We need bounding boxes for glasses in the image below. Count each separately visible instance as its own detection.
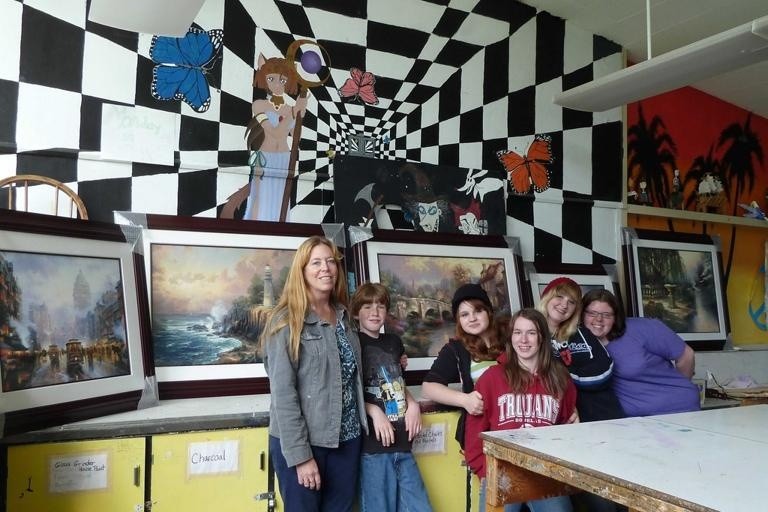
[585,310,613,319]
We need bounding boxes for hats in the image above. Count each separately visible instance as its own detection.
[452,284,489,316]
[542,277,579,296]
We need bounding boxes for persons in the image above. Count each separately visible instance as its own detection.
[349,283,432,511]
[536,278,625,421]
[243,58,309,219]
[464,308,581,512]
[260,237,408,512]
[579,288,700,417]
[422,285,505,454]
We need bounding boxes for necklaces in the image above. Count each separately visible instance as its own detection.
[518,358,539,376]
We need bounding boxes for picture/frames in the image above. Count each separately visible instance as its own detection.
[622,226,734,352]
[0,208,159,434]
[520,262,624,316]
[114,210,350,400]
[348,225,531,387]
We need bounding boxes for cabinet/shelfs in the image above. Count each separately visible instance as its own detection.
[3,435,149,511]
[148,428,273,511]
[470,472,480,511]
[410,410,469,511]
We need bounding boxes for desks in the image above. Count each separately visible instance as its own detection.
[479,402,768,511]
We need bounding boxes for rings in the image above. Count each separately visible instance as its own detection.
[310,480,315,484]
